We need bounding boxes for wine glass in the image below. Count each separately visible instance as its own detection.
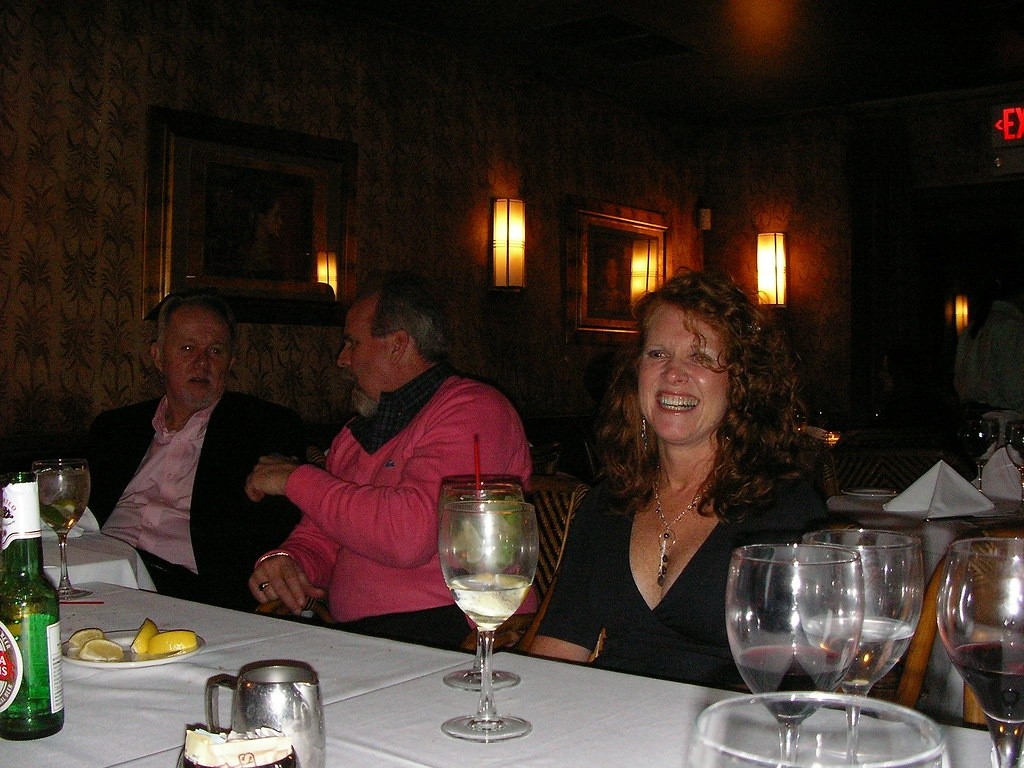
[934,538,1024,767]
[1005,421,1024,518]
[965,418,998,492]
[795,530,921,768]
[32,458,92,599]
[438,501,540,740]
[725,539,864,768]
[442,483,527,689]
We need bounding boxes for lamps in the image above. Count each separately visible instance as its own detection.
[756,231,786,308]
[488,198,530,294]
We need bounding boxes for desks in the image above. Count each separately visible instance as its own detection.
[0,531,994,768]
[821,494,1023,730]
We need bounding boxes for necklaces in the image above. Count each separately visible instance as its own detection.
[645,466,712,589]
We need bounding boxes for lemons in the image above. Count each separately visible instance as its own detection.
[40,499,76,528]
[130,619,197,655]
[450,573,529,615]
[69,628,124,660]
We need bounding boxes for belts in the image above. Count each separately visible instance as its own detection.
[139,549,178,574]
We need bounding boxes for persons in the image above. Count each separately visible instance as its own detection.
[243,286,541,652]
[85,287,305,620]
[526,265,832,698]
[945,270,1024,415]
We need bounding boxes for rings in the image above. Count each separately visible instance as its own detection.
[257,581,272,591]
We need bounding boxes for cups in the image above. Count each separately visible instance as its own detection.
[177,739,297,768]
[687,689,946,767]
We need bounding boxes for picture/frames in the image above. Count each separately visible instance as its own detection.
[140,103,358,328]
[558,190,672,346]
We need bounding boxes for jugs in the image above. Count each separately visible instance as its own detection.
[204,659,328,768]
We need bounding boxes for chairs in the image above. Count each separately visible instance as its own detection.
[462,444,1024,732]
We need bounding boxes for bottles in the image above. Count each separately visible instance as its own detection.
[0,470,64,742]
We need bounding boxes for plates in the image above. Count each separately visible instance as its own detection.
[840,488,898,498]
[61,628,206,669]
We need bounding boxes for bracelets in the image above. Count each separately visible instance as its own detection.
[257,551,290,564]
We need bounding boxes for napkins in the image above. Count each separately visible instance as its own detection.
[886,461,994,521]
